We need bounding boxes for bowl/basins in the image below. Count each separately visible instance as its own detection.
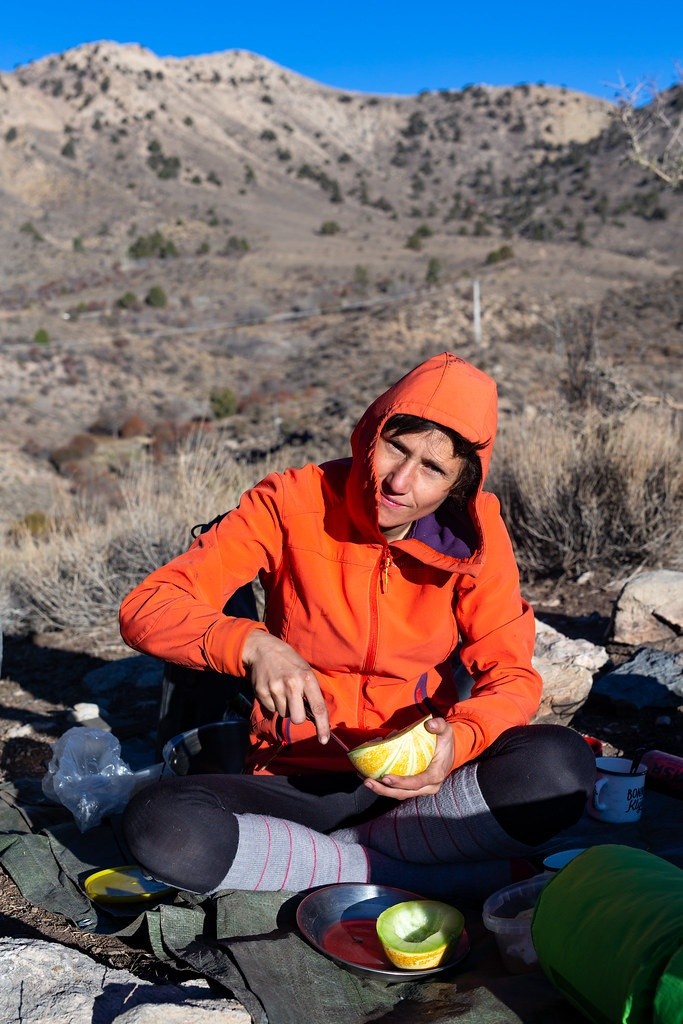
[482,873,553,971]
[162,719,250,776]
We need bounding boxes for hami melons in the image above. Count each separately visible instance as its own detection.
[376,899,470,970]
[348,713,438,781]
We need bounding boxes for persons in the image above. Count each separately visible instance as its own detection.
[119,353,598,896]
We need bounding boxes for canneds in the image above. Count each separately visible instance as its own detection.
[642,749,683,794]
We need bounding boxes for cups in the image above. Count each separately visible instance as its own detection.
[585,757,648,823]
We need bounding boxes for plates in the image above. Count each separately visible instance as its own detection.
[296,883,470,983]
[543,848,586,870]
